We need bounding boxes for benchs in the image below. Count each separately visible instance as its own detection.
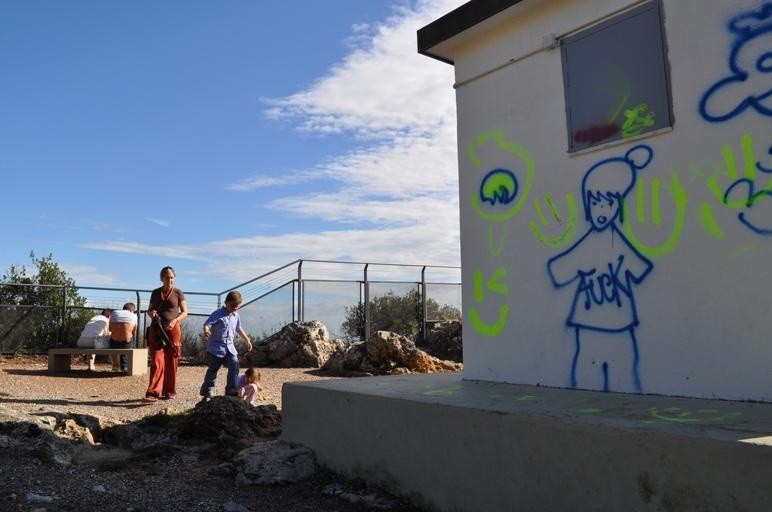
[46,347,149,376]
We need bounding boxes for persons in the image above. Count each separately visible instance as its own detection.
[77,309,113,370]
[200,291,253,401]
[238,368,263,404]
[109,303,138,374]
[142,267,188,401]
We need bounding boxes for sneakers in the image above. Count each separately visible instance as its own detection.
[89,367,128,371]
[142,393,158,402]
[165,394,175,400]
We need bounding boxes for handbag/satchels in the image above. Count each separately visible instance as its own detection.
[146,315,173,349]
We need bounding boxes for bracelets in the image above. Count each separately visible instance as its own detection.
[176,318,178,322]
